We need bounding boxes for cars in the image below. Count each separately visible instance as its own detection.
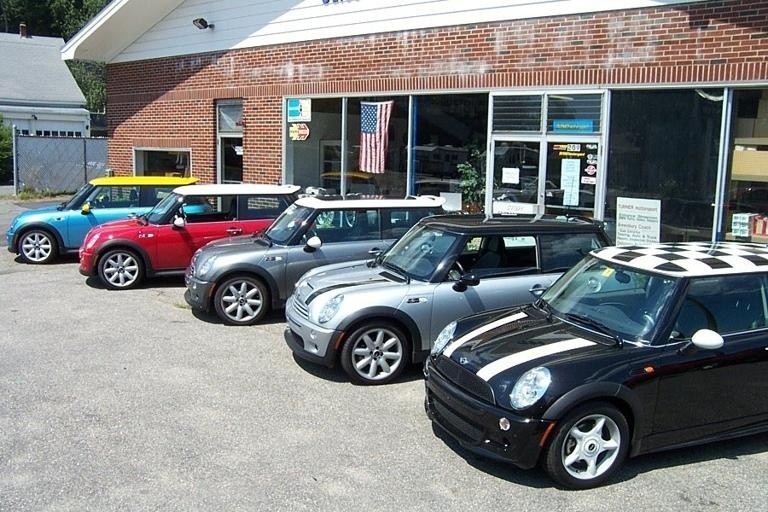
[174,195,445,327]
[422,240,767,491]
[5,176,198,265]
[316,168,573,208]
[283,210,612,386]
[79,184,297,290]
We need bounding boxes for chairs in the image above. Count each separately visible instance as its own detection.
[128,189,139,208]
[476,235,504,269]
[630,279,682,329]
[222,199,237,222]
[342,209,369,242]
[715,281,756,334]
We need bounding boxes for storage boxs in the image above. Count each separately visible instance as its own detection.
[731,137,768,183]
[735,88,768,138]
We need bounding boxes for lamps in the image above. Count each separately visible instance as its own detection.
[193,17,215,30]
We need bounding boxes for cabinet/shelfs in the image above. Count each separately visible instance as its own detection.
[319,140,341,195]
